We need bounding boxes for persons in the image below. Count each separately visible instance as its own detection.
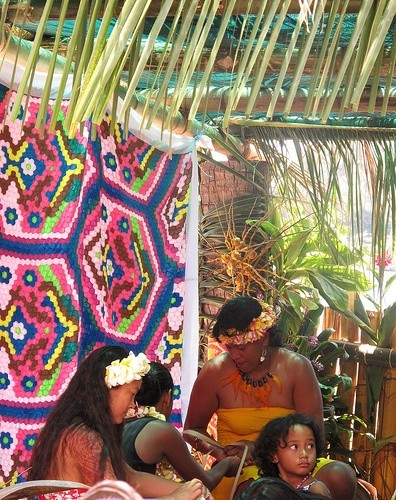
[181,294,358,500]
[120,361,244,492]
[27,344,213,500]
[251,412,332,500]
[235,474,332,500]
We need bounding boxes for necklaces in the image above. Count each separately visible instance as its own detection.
[123,401,176,484]
[295,472,312,490]
[219,348,282,409]
